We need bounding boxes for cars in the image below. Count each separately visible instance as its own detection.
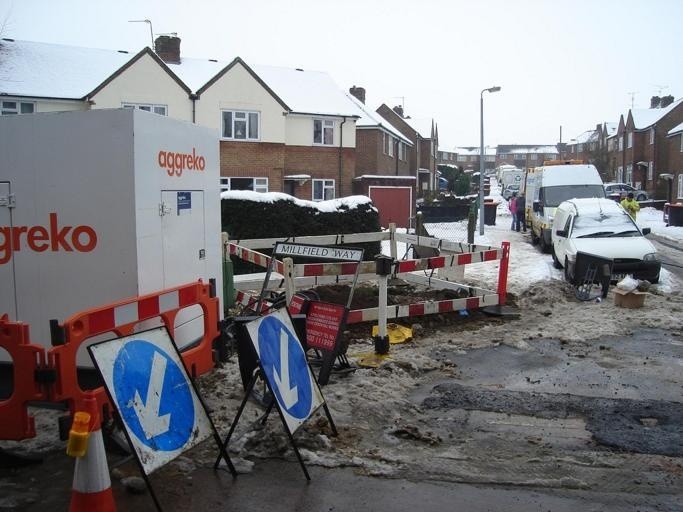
[493,161,524,198]
[600,180,645,201]
[551,198,660,285]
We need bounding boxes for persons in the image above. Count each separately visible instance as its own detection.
[620,193,640,222]
[509,192,517,230]
[516,192,527,232]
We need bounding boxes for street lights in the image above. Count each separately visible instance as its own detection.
[478,84,500,237]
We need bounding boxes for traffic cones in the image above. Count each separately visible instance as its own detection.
[67,391,116,512]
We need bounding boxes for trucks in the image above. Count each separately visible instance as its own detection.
[1,110,220,452]
[525,158,605,252]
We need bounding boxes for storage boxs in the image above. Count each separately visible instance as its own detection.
[610,286,650,307]
